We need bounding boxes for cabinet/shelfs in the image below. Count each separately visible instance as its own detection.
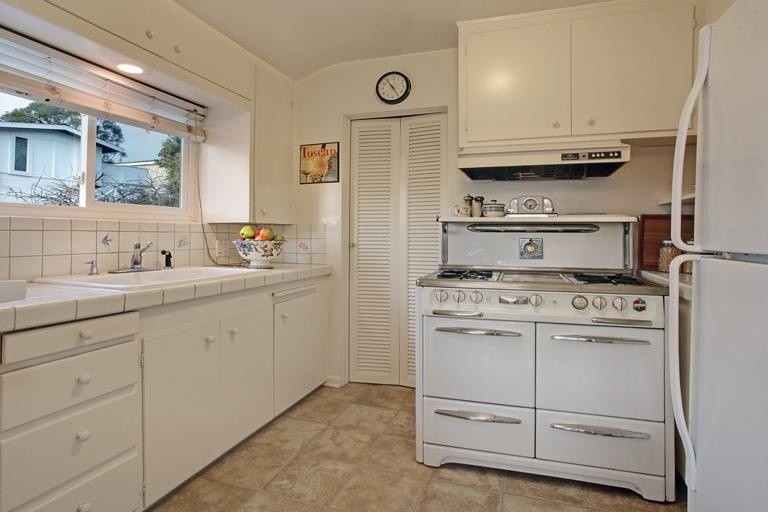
[275,277,332,422]
[458,2,699,147]
[0,311,144,512]
[144,290,276,509]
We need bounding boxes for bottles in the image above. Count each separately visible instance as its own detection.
[458,195,484,218]
[657,240,683,273]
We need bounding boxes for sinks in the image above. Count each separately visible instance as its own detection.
[31,267,256,289]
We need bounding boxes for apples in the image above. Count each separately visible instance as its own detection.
[239,225,284,241]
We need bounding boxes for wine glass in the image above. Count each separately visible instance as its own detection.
[231,240,288,271]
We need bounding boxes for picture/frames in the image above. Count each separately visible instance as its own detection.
[300,141,339,183]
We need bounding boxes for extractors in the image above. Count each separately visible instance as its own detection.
[457,142,633,181]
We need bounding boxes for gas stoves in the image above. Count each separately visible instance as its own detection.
[416,267,663,316]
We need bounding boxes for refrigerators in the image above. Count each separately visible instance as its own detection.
[664,0,768,511]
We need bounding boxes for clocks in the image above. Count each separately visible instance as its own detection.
[375,69,411,104]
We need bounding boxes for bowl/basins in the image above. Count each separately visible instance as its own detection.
[482,199,506,217]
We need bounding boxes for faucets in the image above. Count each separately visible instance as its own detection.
[132,241,154,267]
